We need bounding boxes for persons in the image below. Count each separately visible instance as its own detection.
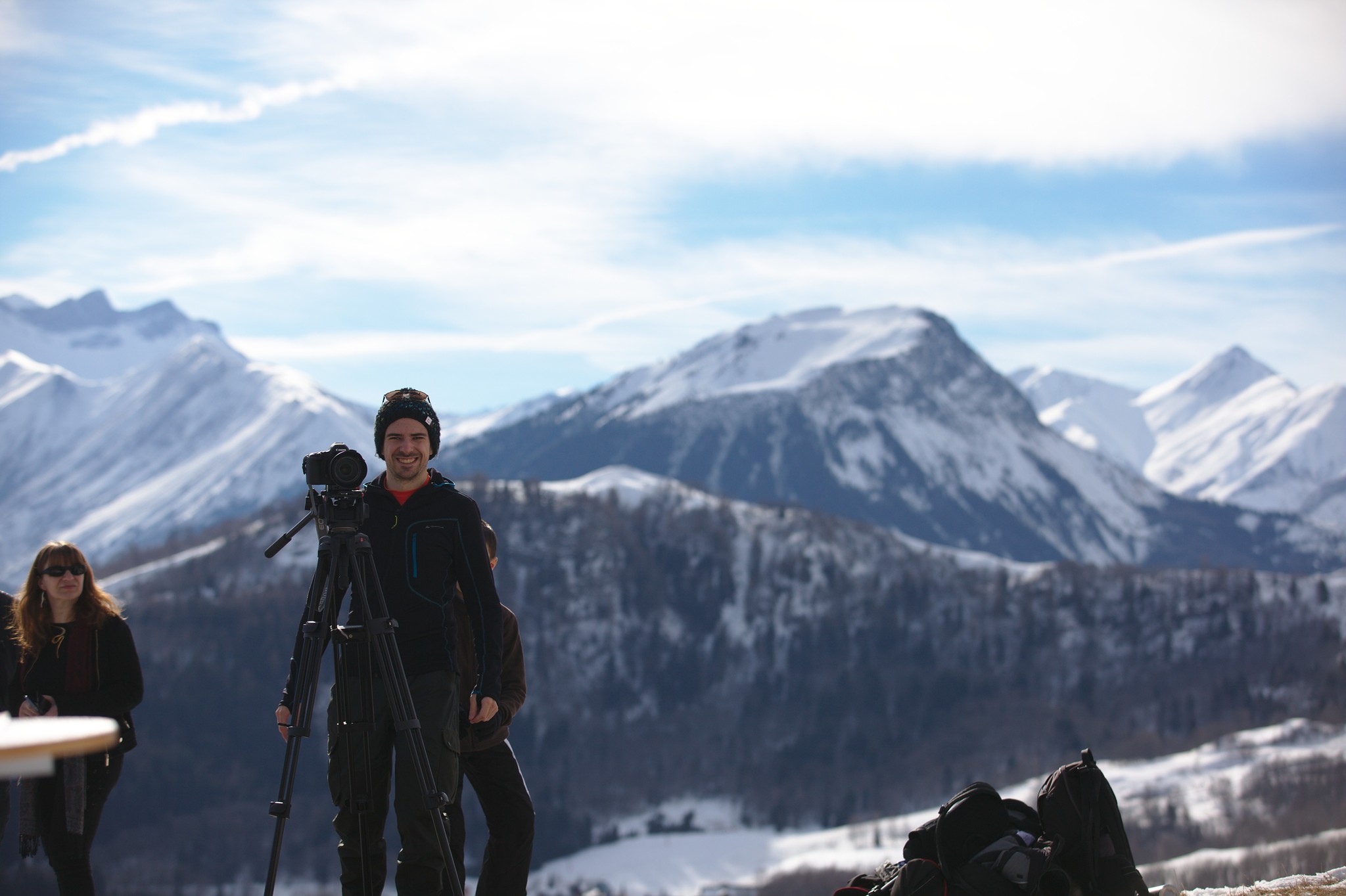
[273,387,510,896]
[414,517,539,896]
[3,539,146,896]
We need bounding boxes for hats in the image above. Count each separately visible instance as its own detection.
[374,387,440,460]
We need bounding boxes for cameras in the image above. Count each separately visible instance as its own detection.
[301,441,368,489]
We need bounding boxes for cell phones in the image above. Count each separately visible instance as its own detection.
[24,690,52,716]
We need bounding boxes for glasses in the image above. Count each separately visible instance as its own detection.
[38,563,85,577]
[382,390,432,408]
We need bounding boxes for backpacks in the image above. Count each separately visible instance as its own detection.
[835,748,1149,896]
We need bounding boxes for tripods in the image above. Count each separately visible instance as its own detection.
[262,492,466,896]
